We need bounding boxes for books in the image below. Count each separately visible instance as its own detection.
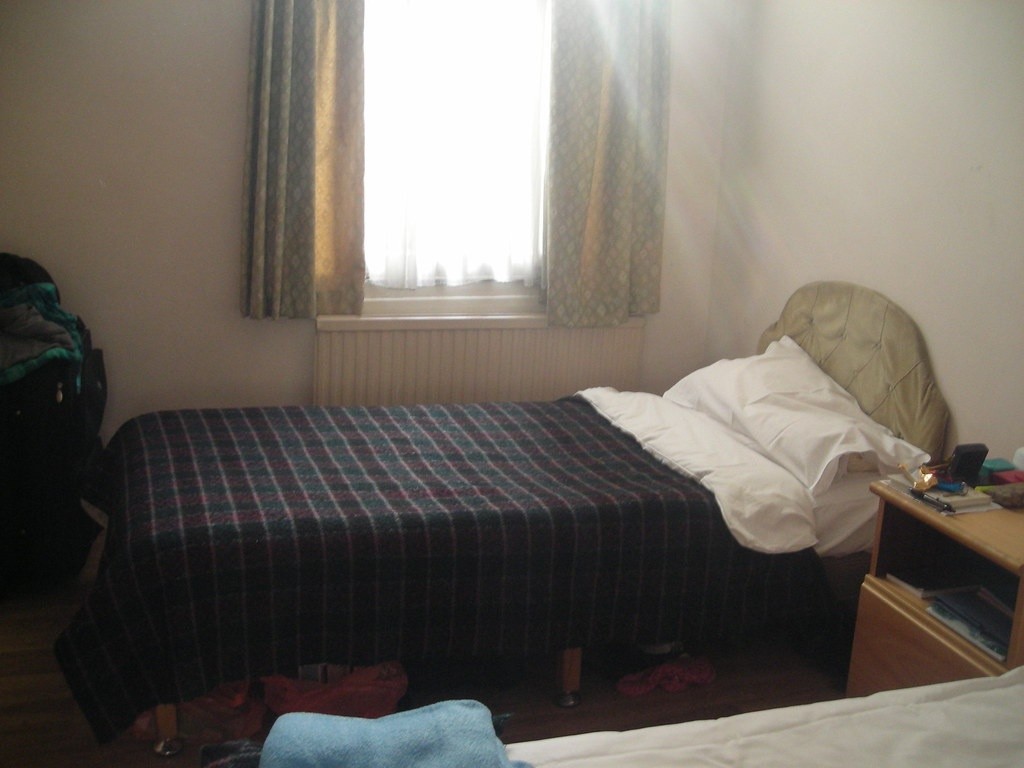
[886,557,989,599]
[924,580,1018,664]
[887,474,991,508]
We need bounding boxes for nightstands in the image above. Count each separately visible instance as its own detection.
[845,478,1024,701]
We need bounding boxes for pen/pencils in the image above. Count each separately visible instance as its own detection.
[908,487,957,513]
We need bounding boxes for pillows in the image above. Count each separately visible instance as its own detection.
[661,336,931,496]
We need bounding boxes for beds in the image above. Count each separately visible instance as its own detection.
[53,281,951,747]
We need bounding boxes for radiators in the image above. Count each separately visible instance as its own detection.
[311,313,645,403]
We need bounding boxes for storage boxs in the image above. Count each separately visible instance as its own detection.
[979,457,1015,485]
[948,444,989,481]
